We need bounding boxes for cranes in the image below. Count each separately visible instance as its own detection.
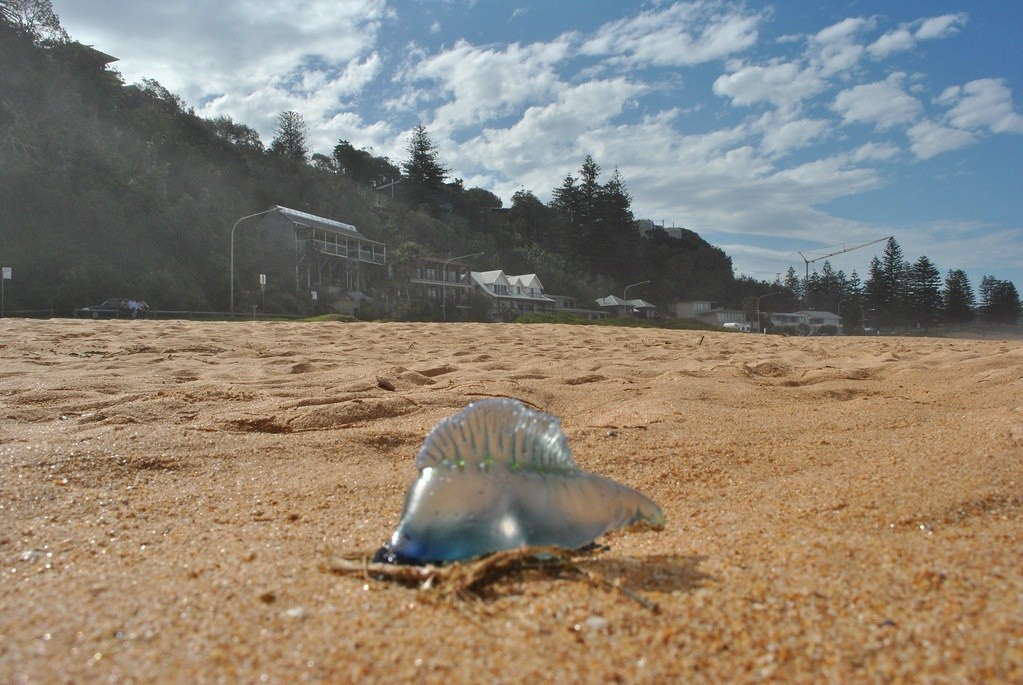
[797,235,892,311]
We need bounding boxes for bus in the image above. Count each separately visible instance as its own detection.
[724,322,753,333]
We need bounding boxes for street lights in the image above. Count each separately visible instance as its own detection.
[230,205,283,314]
[623,279,651,315]
[441,250,487,321]
[756,291,781,331]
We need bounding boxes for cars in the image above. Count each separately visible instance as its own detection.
[75,297,132,318]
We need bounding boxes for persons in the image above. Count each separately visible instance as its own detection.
[138,299,151,319]
[129,296,140,319]
[120,297,132,319]
